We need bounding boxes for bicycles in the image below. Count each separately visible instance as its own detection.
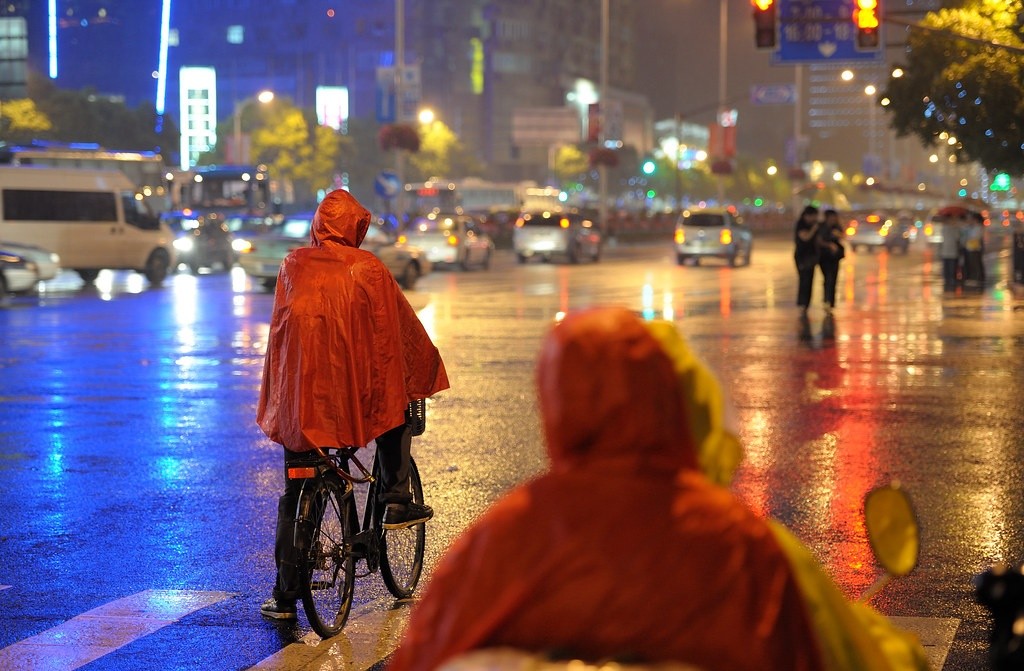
[287,398,425,638]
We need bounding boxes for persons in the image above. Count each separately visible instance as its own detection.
[943,211,984,292]
[257,188,450,618]
[387,306,930,671]
[794,206,844,309]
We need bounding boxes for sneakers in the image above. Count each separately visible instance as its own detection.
[384,502,433,529]
[262,599,296,618]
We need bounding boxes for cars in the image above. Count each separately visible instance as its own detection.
[511,212,602,264]
[844,212,943,254]
[0,241,59,299]
[159,212,432,290]
[396,216,495,272]
[674,207,752,268]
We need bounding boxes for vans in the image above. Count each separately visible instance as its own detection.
[0,182,174,284]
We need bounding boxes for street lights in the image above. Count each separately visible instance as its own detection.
[232,88,274,163]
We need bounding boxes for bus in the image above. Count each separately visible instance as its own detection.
[0,147,276,239]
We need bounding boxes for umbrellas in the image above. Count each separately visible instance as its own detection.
[939,196,991,214]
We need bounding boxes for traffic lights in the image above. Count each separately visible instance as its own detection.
[853,0,881,53]
[993,173,1010,201]
[642,159,659,179]
[753,0,776,48]
